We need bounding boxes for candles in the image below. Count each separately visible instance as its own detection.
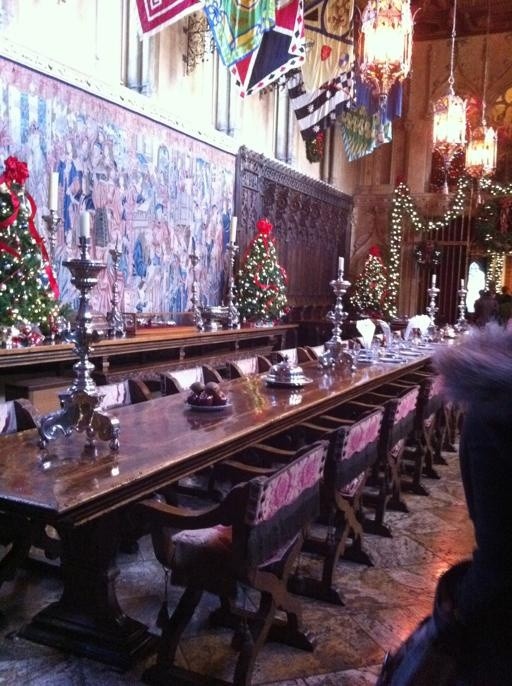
[460,278,465,287]
[229,213,238,243]
[48,167,60,210]
[80,209,91,240]
[431,272,437,284]
[338,254,345,272]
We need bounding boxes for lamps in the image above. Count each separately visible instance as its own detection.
[428,0,497,180]
[360,0,418,109]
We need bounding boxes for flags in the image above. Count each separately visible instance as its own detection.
[134,0,415,164]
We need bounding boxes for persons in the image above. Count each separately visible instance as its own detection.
[471,284,511,329]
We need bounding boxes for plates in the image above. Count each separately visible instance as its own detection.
[183,401,233,411]
[347,325,458,365]
[260,374,314,387]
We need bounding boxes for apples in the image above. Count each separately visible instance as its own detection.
[187,381,229,407]
